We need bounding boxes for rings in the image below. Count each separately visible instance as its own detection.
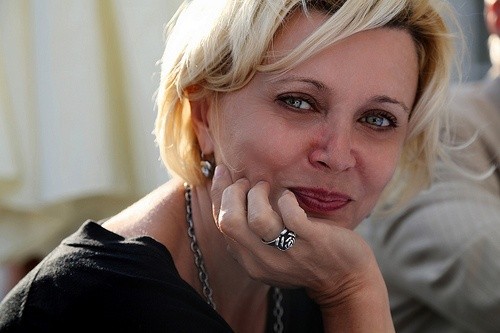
[260,225,297,253]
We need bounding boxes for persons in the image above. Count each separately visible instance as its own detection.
[347,0,498,332]
[0,0,464,333]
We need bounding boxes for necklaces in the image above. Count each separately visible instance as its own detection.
[181,182,284,333]
